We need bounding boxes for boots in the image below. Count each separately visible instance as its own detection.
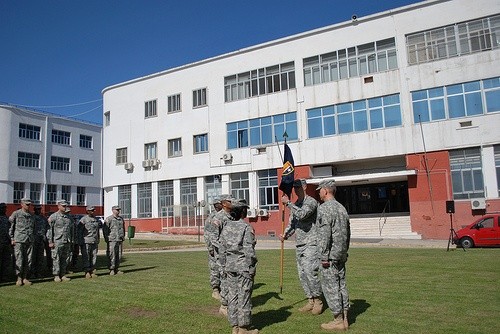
[312,297,323,315]
[212,288,220,300]
[343,310,349,330]
[231,325,239,334]
[219,305,229,316]
[238,326,259,334]
[321,314,345,330]
[297,298,315,312]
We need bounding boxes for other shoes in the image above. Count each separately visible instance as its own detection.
[109,269,123,275]
[85,271,98,279]
[54,275,71,282]
[15,276,32,286]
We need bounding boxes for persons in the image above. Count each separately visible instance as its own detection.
[46,199,75,282]
[203,194,248,317]
[0,203,80,281]
[219,200,259,334]
[279,178,323,316]
[7,197,37,285]
[103,206,125,276]
[76,205,100,278]
[316,179,350,330]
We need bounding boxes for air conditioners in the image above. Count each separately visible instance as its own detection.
[470,198,487,210]
[223,152,232,161]
[142,160,150,168]
[246,209,258,218]
[124,163,133,171]
[257,209,269,217]
[150,158,160,167]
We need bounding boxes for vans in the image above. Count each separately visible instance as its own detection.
[452,214,500,249]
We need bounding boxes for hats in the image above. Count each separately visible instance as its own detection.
[65,208,71,212]
[86,206,95,211]
[231,198,250,209]
[57,199,68,206]
[112,206,121,210]
[21,198,31,205]
[34,205,42,209]
[213,198,222,205]
[0,202,7,208]
[293,178,306,187]
[220,194,234,202]
[316,179,336,190]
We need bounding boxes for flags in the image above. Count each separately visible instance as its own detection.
[279,142,295,200]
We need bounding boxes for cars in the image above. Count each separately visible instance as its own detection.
[95,216,105,227]
